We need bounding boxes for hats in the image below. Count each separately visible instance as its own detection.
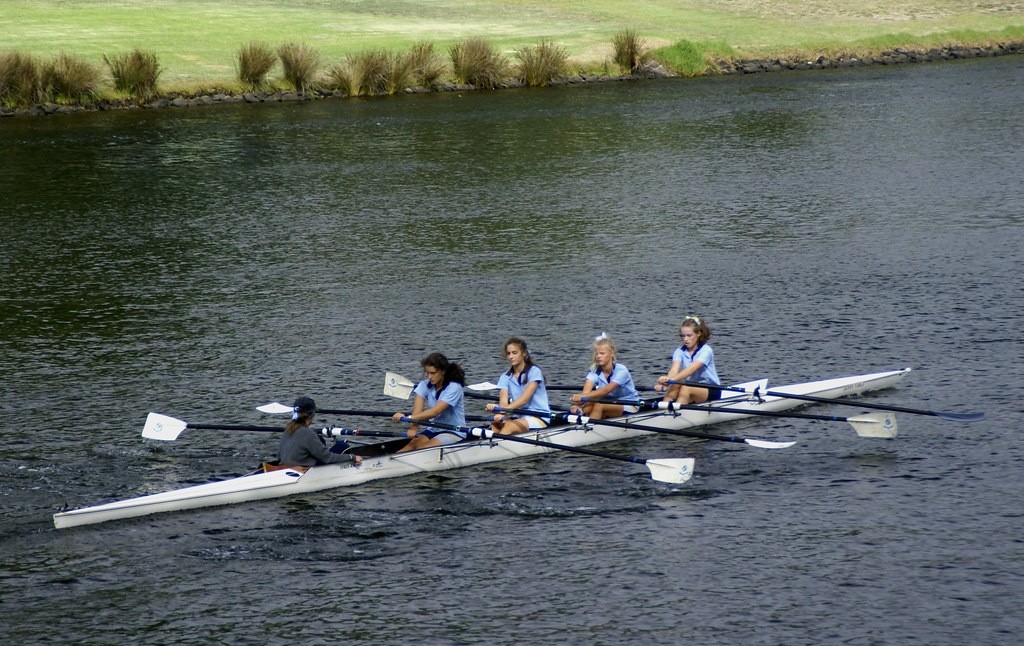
[294,396,315,413]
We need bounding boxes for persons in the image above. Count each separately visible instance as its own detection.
[485,337,551,435]
[570,332,640,420]
[392,352,467,453]
[278,396,363,467]
[653,316,721,405]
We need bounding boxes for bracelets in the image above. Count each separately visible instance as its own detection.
[409,415,412,418]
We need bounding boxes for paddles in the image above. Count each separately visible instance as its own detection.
[669,379,985,422]
[494,405,797,450]
[421,416,696,485]
[463,375,655,398]
[140,410,410,448]
[588,397,899,441]
[378,371,492,412]
[255,402,475,438]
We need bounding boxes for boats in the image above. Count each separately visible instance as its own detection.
[52,366,912,530]
